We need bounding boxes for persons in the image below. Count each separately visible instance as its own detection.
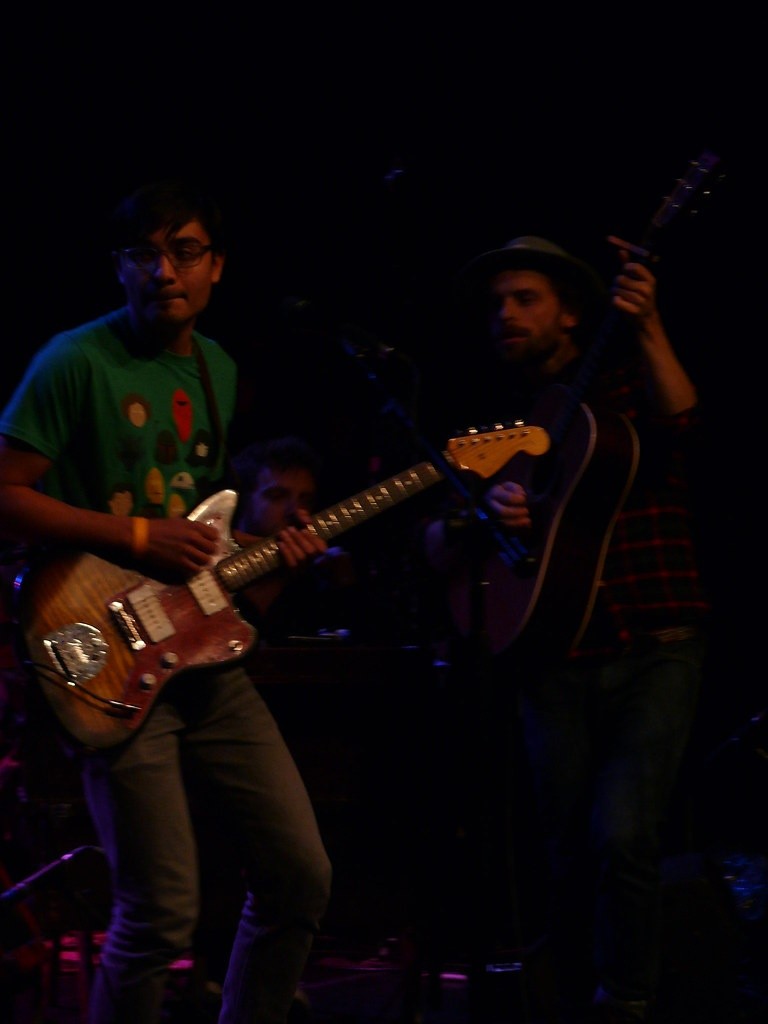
[0,192,365,1019]
[424,222,703,1023]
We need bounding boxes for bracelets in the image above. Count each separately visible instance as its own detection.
[133,517,149,554]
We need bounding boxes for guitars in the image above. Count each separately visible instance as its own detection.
[393,140,744,690]
[9,409,576,764]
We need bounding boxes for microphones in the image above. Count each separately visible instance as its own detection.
[285,297,394,359]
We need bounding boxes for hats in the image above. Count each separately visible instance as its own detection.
[468,235,604,308]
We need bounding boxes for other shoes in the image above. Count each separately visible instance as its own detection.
[609,1005,645,1024]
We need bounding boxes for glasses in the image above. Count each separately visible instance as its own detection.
[122,243,212,270]
